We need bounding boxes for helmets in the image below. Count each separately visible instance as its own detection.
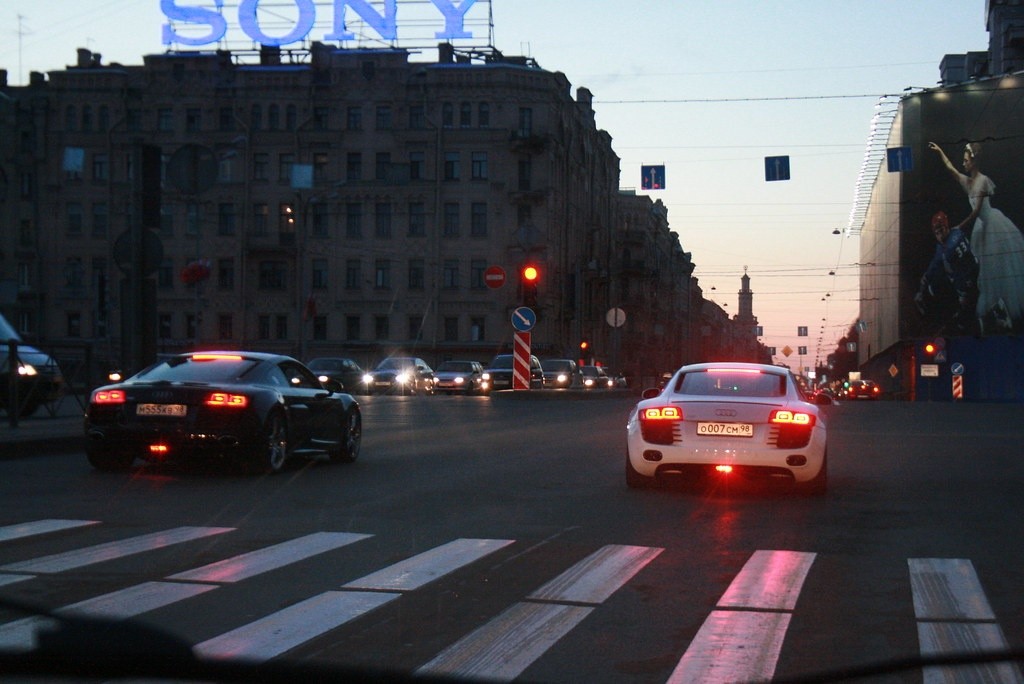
[931,211,948,233]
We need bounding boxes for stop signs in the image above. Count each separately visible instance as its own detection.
[483,265,507,289]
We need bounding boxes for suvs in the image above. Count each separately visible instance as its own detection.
[482,353,545,396]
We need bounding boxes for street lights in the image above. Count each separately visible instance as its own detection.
[298,180,348,363]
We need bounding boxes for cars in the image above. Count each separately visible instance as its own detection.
[539,358,577,390]
[579,365,627,390]
[798,375,881,406]
[363,356,436,396]
[427,360,483,396]
[306,356,365,395]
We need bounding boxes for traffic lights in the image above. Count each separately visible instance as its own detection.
[521,257,541,284]
[926,343,937,356]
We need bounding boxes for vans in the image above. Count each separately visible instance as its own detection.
[0,314,68,421]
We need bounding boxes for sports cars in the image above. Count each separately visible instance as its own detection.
[82,350,363,477]
[624,361,833,496]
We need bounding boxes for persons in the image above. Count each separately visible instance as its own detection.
[927,138,1024,331]
[914,210,1014,337]
